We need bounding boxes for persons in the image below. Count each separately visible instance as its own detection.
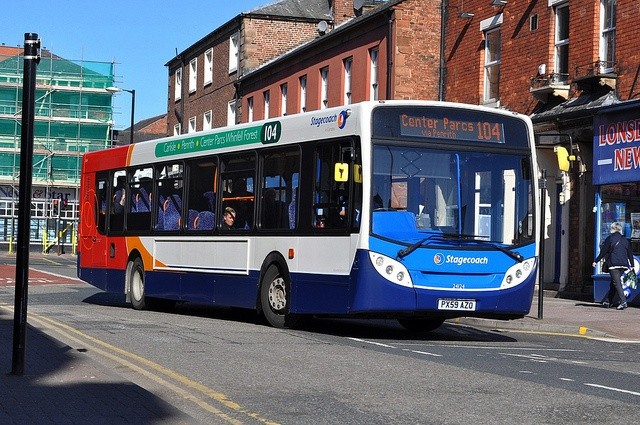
[222,208,239,233]
[593,221,635,310]
[260,189,285,229]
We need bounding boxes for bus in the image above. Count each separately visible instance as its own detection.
[76,99,541,329]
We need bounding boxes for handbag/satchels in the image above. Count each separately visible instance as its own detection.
[601,235,624,271]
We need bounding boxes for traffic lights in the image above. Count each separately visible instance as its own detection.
[54,200,58,207]
[62,199,67,205]
